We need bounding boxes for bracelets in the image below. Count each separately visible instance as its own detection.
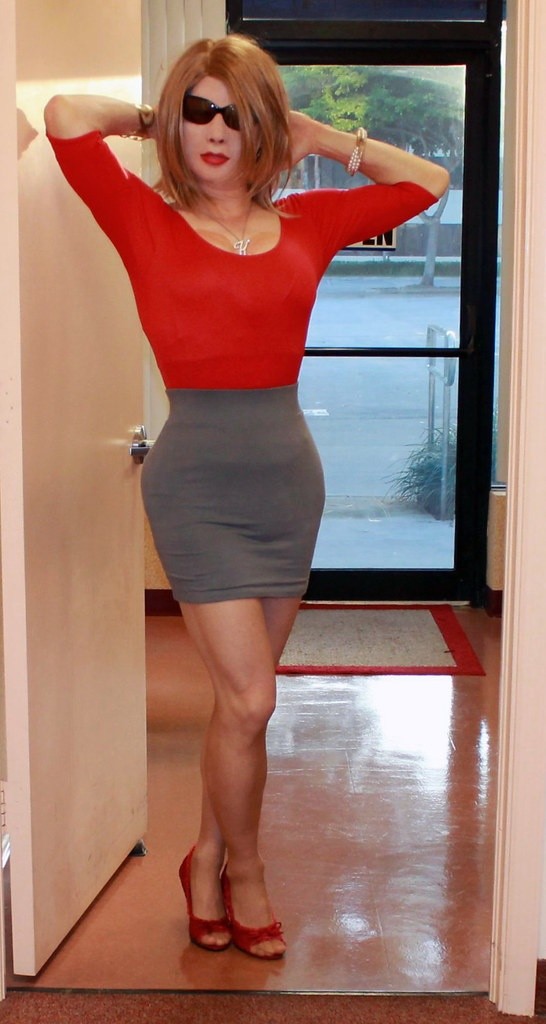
[345,126,368,177]
[119,103,142,139]
[135,102,155,140]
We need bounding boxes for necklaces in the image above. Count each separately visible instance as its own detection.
[200,203,252,256]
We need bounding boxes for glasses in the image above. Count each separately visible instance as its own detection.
[182,93,260,131]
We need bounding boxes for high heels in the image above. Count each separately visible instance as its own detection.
[221,862,287,960]
[178,845,232,952]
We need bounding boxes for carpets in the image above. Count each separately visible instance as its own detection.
[275,604,485,676]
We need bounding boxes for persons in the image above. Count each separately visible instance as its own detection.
[44,36,449,961]
